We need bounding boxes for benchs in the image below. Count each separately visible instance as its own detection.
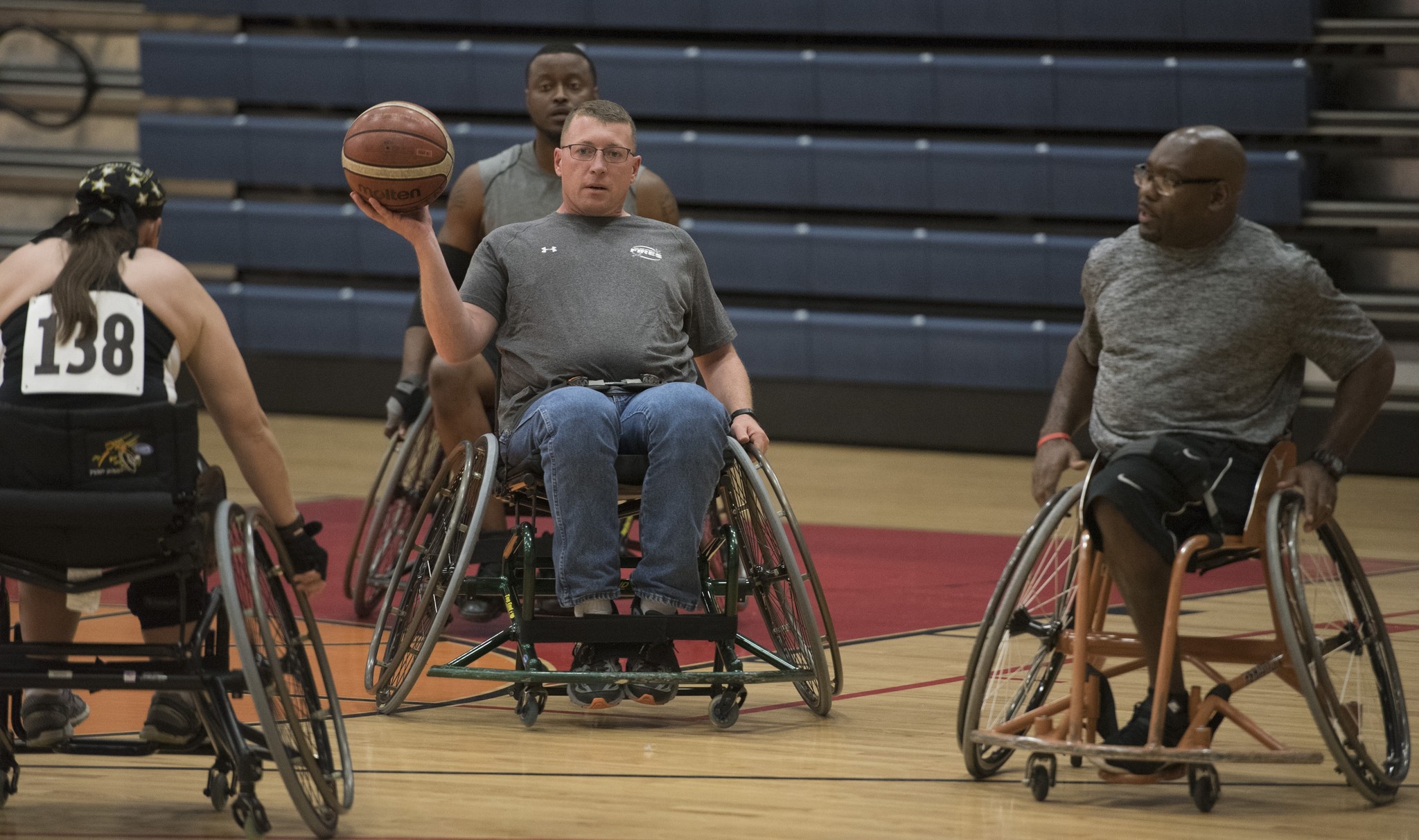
[138,0,1317,397]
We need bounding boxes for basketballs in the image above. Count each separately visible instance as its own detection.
[341,101,455,213]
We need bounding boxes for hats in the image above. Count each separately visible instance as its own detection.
[73,161,166,219]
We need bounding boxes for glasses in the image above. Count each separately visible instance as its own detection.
[1131,163,1226,195]
[560,144,637,163]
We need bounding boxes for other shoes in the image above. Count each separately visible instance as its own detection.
[458,563,506,619]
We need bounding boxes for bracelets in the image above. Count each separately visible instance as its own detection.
[728,407,758,424]
[1036,432,1072,448]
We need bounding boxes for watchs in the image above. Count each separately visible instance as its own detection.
[1309,447,1347,479]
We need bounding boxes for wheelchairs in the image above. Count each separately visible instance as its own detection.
[343,353,844,729]
[0,398,355,840]
[955,416,1412,813]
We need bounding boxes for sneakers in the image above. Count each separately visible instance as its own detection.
[623,595,682,706]
[566,599,625,709]
[18,687,89,747]
[136,690,200,748]
[1082,685,1191,773]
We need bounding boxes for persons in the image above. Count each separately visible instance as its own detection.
[1,163,328,745]
[348,43,770,708]
[1027,125,1398,777]
